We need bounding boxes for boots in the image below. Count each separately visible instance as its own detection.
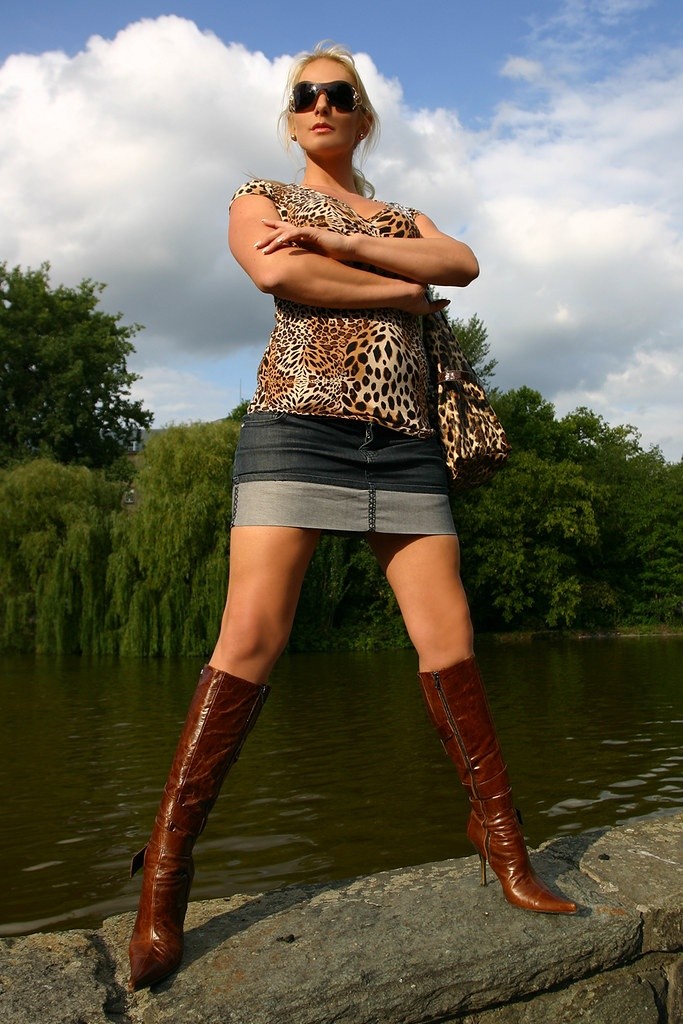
[126,664,269,988]
[418,655,578,916]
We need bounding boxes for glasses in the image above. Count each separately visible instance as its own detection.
[289,80,363,114]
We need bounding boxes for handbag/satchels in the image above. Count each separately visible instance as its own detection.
[422,284,511,494]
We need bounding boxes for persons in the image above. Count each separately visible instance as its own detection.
[122,46,576,993]
